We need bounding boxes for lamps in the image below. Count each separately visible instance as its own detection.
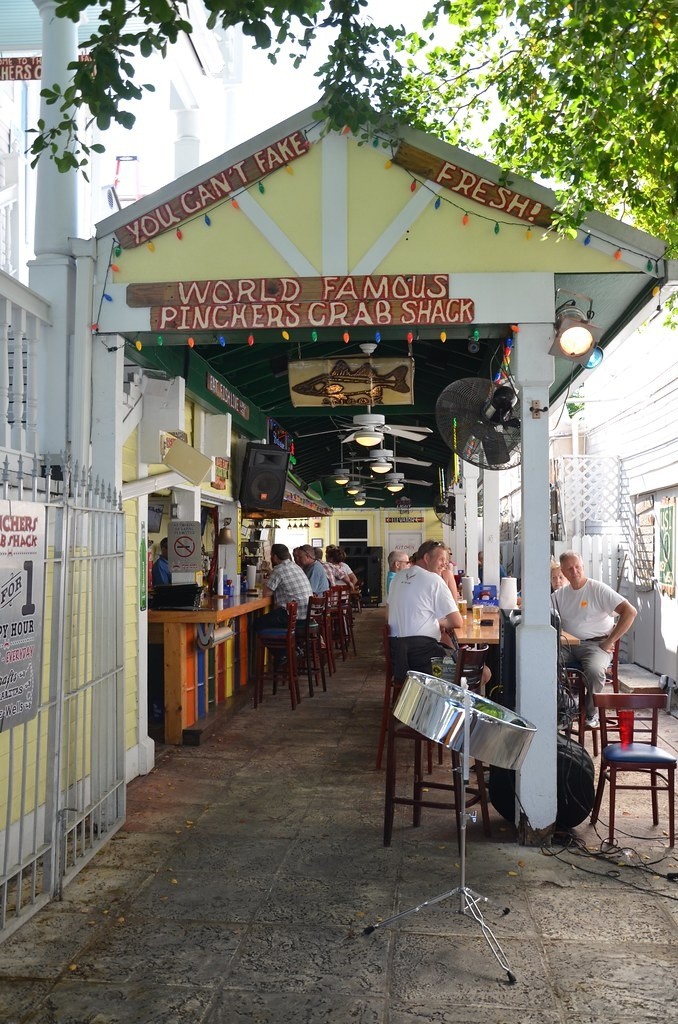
[369,462,393,473]
[548,289,605,370]
[354,499,366,506]
[334,476,349,484]
[354,432,385,447]
[387,483,404,492]
[347,487,359,495]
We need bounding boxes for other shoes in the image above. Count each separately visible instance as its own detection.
[296,657,304,668]
[274,663,289,673]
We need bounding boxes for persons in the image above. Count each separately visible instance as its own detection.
[478,551,505,584]
[254,543,358,665]
[152,537,171,586]
[387,542,492,691]
[551,550,637,728]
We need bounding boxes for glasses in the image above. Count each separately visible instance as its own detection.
[427,541,441,553]
[298,546,314,559]
[399,561,410,564]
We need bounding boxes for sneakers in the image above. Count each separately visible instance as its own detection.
[584,713,599,729]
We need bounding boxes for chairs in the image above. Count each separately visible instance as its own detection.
[590,692,677,847]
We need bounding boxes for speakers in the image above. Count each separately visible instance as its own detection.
[239,442,291,510]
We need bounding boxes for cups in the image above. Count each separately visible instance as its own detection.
[457,600,467,619]
[473,605,483,625]
[618,710,634,745]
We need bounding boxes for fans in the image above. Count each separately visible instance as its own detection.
[435,377,522,470]
[433,491,456,531]
[297,343,433,500]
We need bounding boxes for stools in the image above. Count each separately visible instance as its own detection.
[251,579,622,847]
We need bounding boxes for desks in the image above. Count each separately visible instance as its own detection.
[455,608,580,647]
